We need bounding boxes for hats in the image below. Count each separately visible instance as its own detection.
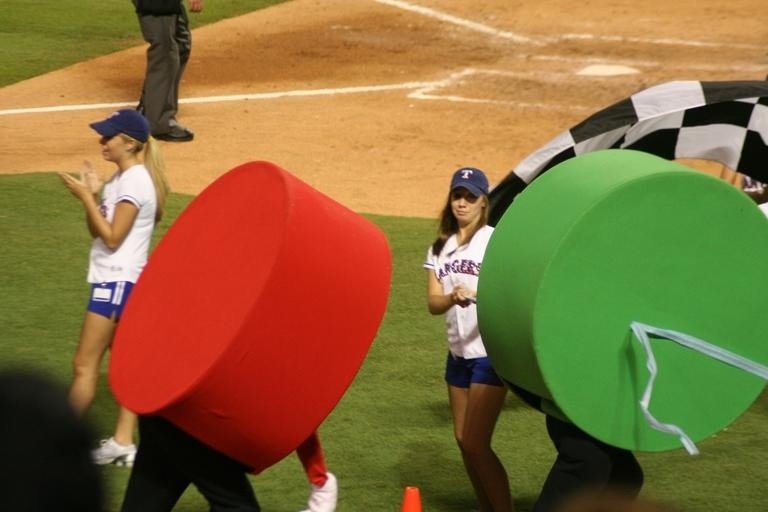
[87,108,150,143]
[450,167,489,198]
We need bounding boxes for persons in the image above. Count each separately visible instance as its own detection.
[128,1,204,142]
[54,108,171,467]
[421,166,516,510]
[118,406,261,511]
[0,366,114,510]
[536,413,644,508]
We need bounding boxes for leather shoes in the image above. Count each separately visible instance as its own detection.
[152,119,194,142]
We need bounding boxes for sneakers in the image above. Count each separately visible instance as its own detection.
[90,436,138,469]
